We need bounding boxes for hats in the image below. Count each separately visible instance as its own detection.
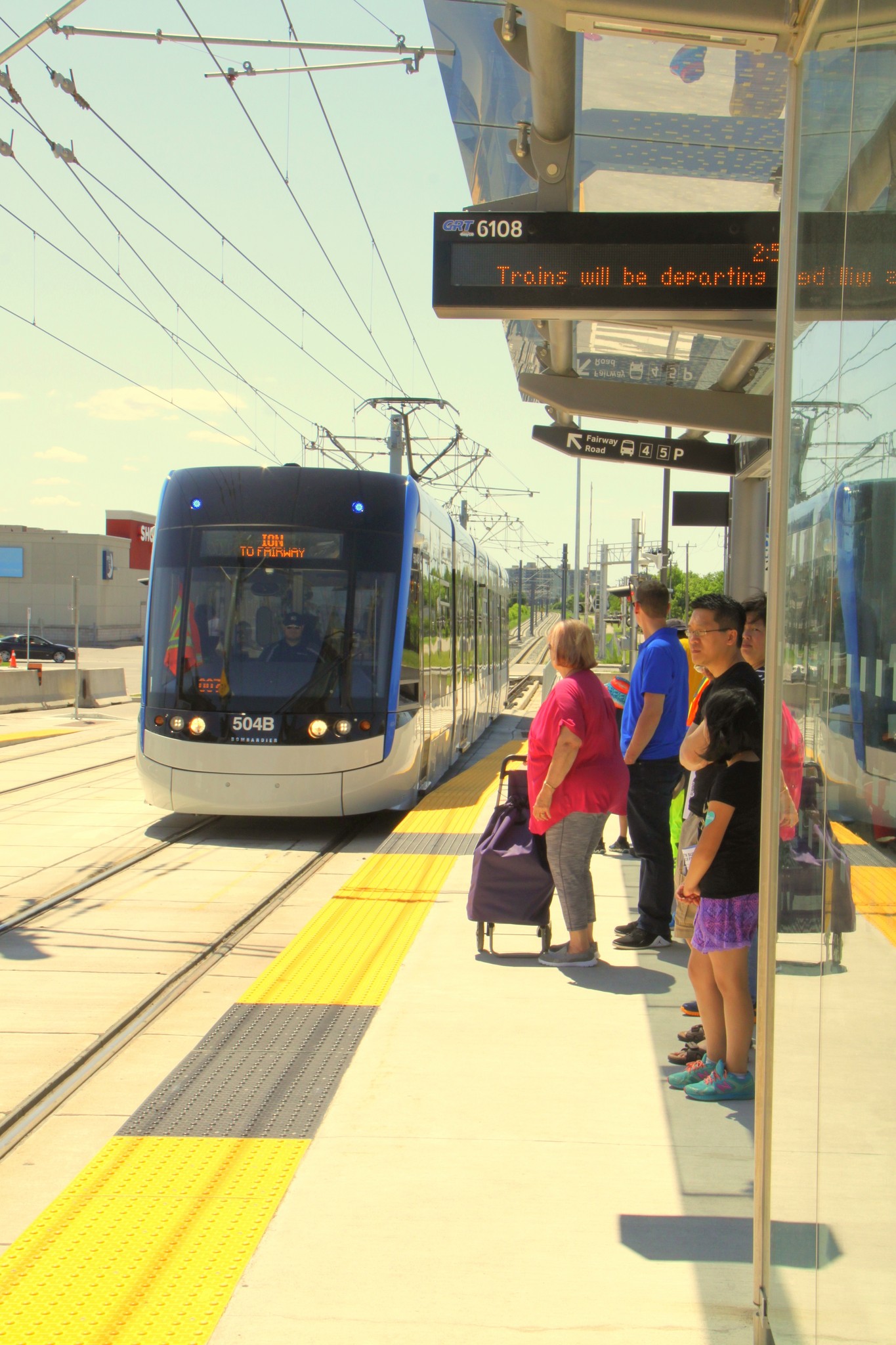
[283,612,304,627]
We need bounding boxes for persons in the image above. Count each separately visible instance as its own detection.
[667,593,764,1066]
[259,611,331,664]
[613,578,690,950]
[780,700,805,854]
[527,619,630,965]
[590,674,631,854]
[674,595,768,1024]
[665,619,704,785]
[668,685,756,1102]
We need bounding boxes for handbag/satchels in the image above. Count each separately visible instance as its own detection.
[466,793,554,926]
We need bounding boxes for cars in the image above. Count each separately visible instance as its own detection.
[0,633,77,663]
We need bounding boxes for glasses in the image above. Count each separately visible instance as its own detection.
[684,628,732,637]
[286,625,301,630]
[632,601,642,607]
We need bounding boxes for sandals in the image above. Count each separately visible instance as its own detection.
[677,1025,706,1043]
[668,1042,707,1065]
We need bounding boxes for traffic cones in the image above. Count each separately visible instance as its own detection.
[8,650,19,668]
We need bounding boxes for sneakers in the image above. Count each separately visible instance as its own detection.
[614,919,639,936]
[667,1053,716,1089]
[683,1059,755,1101]
[612,928,672,950]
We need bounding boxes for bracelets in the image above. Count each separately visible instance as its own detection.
[780,786,788,794]
[544,781,557,790]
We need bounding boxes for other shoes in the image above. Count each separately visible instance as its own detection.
[538,946,597,967]
[548,940,601,958]
[681,1001,700,1017]
[669,911,675,931]
[753,1007,757,1024]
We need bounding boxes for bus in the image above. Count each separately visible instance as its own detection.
[139,392,513,817]
